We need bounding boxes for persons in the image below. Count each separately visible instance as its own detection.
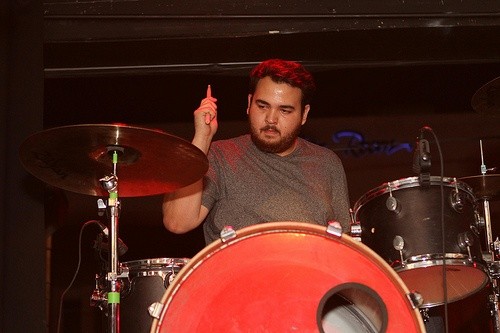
[163,58,351,249]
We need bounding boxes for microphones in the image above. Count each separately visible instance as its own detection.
[407,128,426,176]
[96,220,128,257]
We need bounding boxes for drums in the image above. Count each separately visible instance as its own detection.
[148,220,426,333]
[118,257,193,333]
[350,175,490,309]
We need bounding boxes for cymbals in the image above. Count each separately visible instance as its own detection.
[471,75,500,110]
[19,123,209,198]
